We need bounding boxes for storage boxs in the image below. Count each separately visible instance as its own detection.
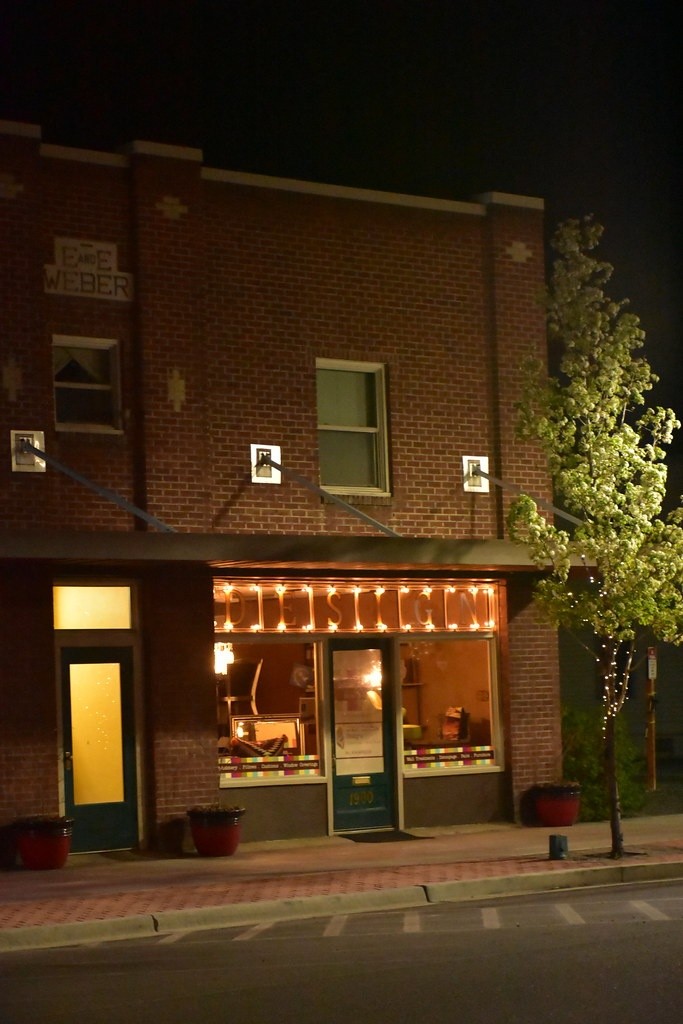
[304,721,317,755]
[299,697,316,721]
[438,711,470,741]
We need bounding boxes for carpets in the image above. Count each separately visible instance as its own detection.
[337,829,435,843]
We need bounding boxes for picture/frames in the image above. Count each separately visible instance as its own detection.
[229,713,302,754]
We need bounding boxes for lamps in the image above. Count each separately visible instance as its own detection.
[214,642,235,676]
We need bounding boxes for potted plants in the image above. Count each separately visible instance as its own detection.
[9,814,77,870]
[529,778,581,828]
[187,801,246,858]
